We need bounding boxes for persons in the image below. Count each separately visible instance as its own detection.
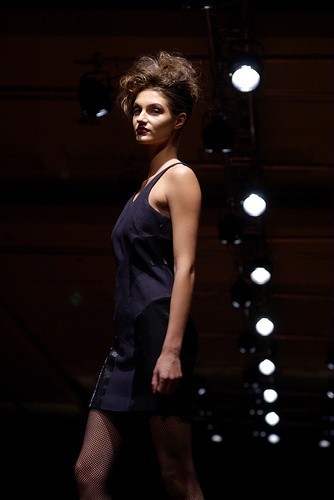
[72,50,206,496]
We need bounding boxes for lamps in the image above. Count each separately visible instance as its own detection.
[217,40,282,454]
